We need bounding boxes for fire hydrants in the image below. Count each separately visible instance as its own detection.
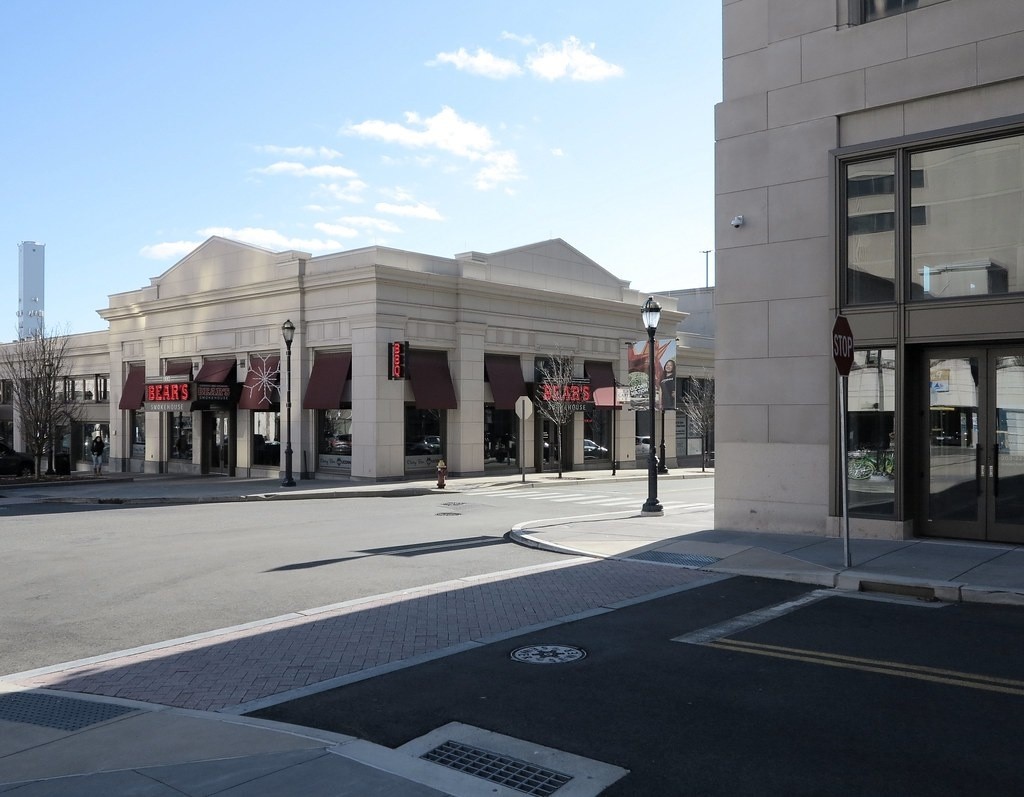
[435,459,446,487]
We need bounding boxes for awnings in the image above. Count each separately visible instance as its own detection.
[406,350,619,410]
[165,352,353,410]
[119,365,146,410]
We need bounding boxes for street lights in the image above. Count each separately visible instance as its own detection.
[638,297,665,517]
[43,359,56,476]
[281,319,297,487]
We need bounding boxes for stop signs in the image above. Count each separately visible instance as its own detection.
[832,315,854,375]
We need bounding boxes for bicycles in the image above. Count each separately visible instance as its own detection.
[845,446,897,481]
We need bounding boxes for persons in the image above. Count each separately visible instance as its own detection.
[659,360,675,408]
[90,436,106,475]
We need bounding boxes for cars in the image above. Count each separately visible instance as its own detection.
[934,427,970,446]
[216,433,266,463]
[410,436,442,454]
[585,439,608,459]
[635,435,652,456]
[0,442,36,479]
[266,441,280,460]
[332,434,352,456]
[490,434,558,463]
